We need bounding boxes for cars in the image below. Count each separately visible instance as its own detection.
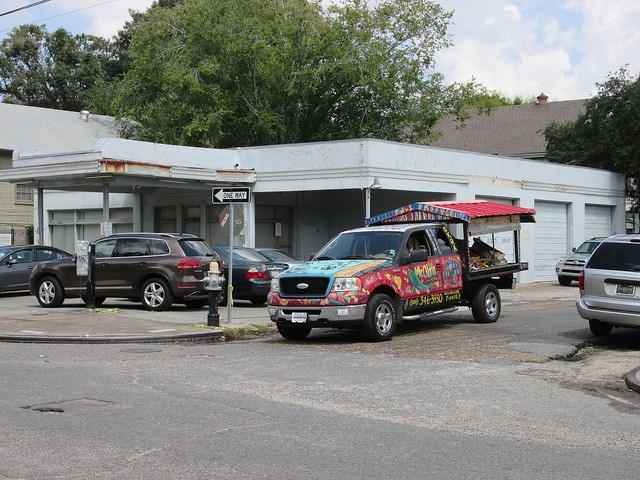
[0,245,74,293]
[253,248,307,268]
[211,245,289,307]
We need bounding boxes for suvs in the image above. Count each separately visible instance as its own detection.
[556,236,610,285]
[29,233,224,311]
[576,233,640,335]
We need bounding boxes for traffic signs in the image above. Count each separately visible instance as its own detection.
[211,187,251,204]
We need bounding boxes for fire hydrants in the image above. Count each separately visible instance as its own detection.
[202,257,226,326]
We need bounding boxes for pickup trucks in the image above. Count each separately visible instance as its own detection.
[267,200,529,342]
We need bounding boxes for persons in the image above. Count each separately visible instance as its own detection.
[407,237,416,255]
